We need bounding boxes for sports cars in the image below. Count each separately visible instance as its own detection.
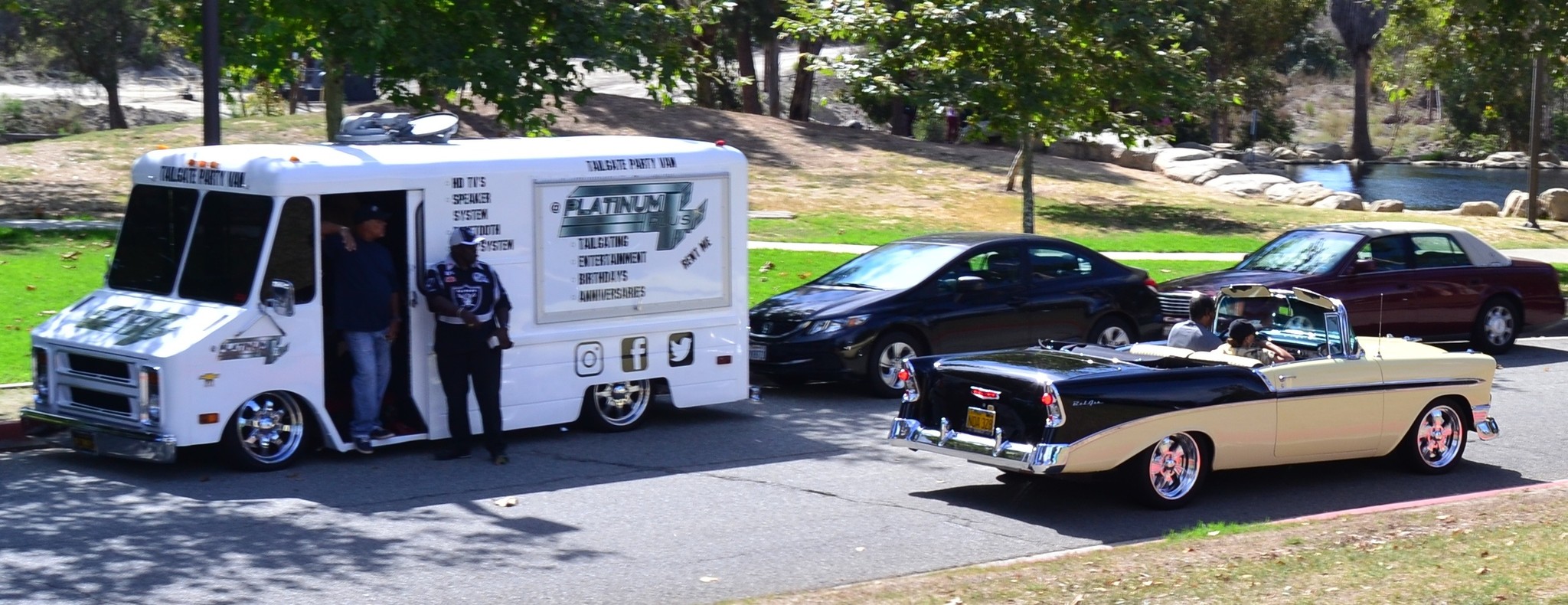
[887,287,1500,512]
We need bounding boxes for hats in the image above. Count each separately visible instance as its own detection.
[358,201,392,224]
[1227,319,1259,338]
[447,227,486,251]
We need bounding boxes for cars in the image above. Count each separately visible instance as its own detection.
[1156,224,1567,356]
[750,232,1165,398]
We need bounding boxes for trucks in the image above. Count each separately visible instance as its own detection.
[20,111,766,475]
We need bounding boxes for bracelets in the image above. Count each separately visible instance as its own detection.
[339,227,350,230]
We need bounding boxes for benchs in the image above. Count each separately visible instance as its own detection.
[1411,250,1469,268]
[1130,342,1266,369]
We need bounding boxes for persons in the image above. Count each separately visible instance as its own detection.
[1211,319,1296,366]
[1168,295,1223,352]
[426,226,513,465]
[322,201,400,454]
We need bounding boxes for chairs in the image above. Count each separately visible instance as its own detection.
[1027,254,1081,276]
[987,252,1028,275]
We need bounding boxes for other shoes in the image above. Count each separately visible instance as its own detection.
[432,446,471,460]
[352,436,373,454]
[493,453,511,466]
[370,428,396,439]
